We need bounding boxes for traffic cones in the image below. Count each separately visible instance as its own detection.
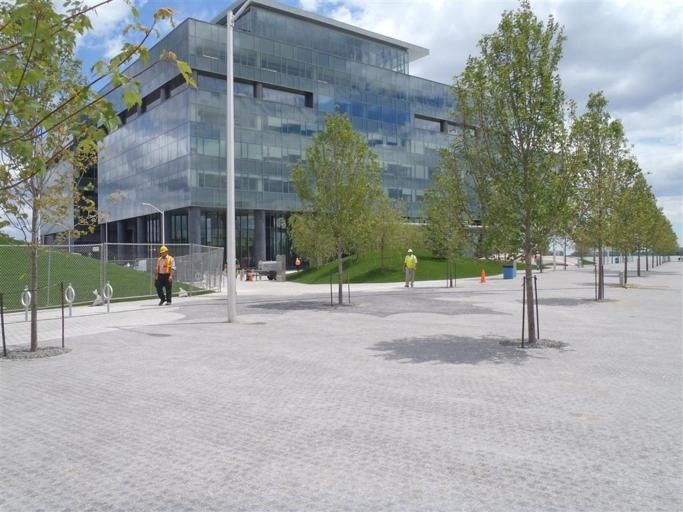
[481,272,485,283]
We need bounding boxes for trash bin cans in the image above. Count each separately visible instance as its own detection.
[504,265,513,279]
[247,270,253,282]
[615,259,618,264]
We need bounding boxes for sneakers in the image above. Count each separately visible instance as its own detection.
[157,298,172,306]
[404,282,414,288]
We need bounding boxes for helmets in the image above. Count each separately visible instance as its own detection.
[407,248,413,253]
[159,246,169,254]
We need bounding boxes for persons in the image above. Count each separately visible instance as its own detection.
[293,256,300,272]
[403,248,417,288]
[153,244,177,306]
[533,252,543,270]
